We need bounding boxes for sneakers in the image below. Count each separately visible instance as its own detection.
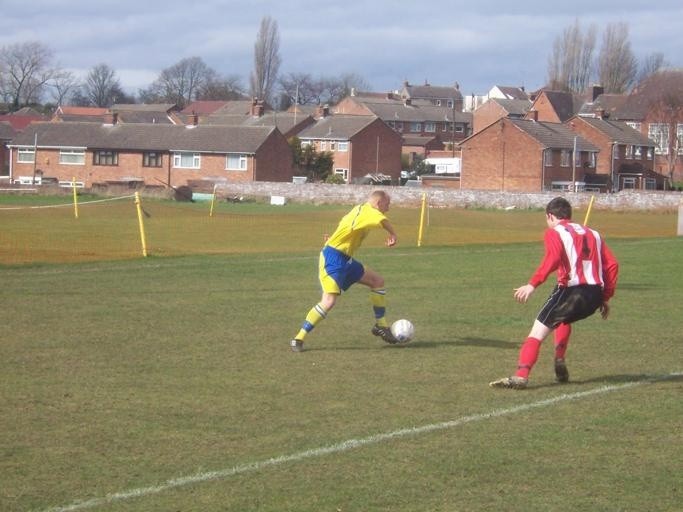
[555,361,569,383]
[488,376,528,390]
[290,338,305,352]
[371,323,398,345]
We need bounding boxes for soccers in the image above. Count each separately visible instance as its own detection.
[391,319,415,343]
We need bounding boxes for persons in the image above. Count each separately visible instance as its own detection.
[291,189,401,355]
[489,195,618,389]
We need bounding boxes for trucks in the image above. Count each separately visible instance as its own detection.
[407,156,459,175]
